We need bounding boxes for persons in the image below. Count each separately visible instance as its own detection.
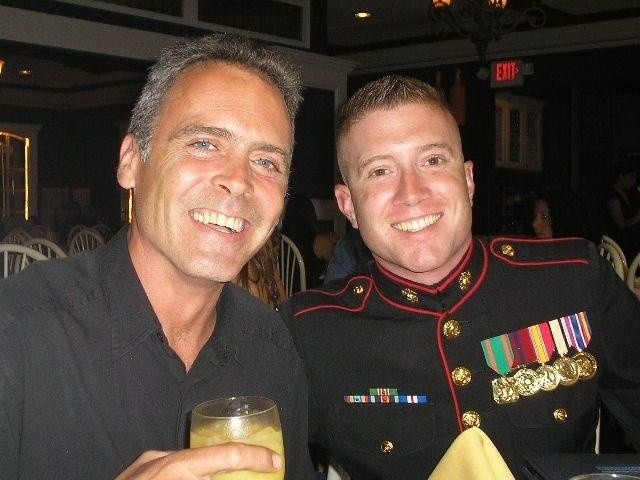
[276,192,339,294]
[601,159,639,274]
[274,74,639,480]
[1,30,322,480]
[529,190,589,242]
[233,205,289,308]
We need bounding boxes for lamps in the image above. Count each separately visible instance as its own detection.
[428,0,547,69]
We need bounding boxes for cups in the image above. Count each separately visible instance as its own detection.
[187,394,285,480]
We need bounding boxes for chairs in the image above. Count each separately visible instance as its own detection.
[598,233,640,301]
[0,223,116,282]
[276,233,308,299]
